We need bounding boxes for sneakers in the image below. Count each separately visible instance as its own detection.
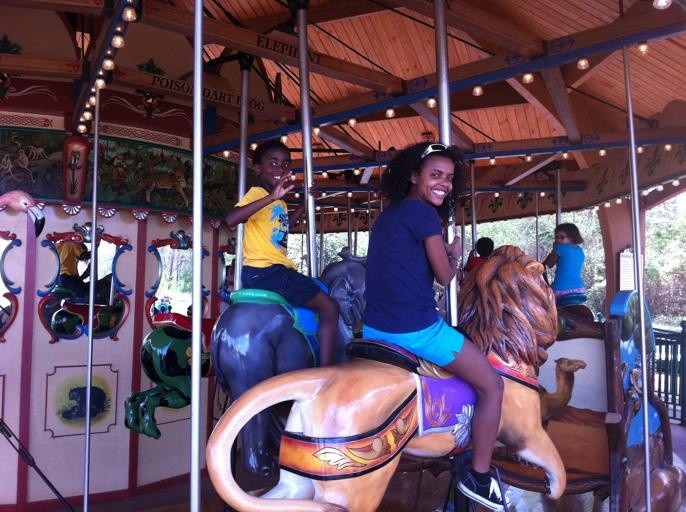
[457,469,510,511]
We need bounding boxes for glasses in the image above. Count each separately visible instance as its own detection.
[418,143,451,159]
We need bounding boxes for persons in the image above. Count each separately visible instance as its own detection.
[467,236,494,272]
[225,138,341,369]
[542,222,586,300]
[56,241,91,293]
[357,140,511,510]
